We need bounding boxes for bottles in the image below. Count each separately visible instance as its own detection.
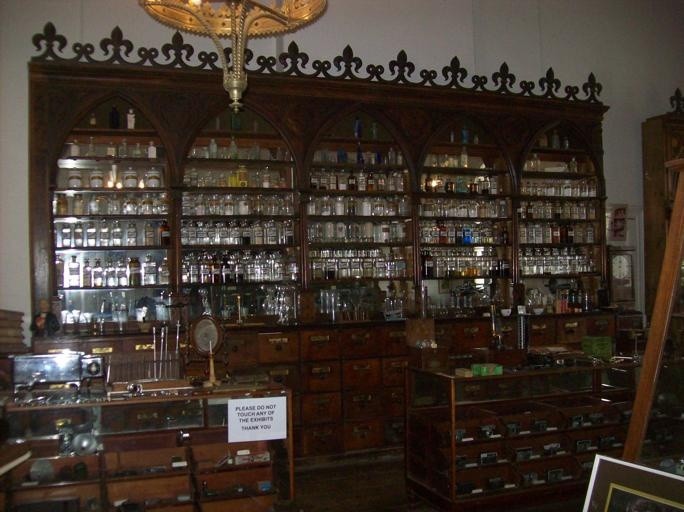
[51,103,609,336]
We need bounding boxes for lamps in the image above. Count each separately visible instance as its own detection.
[136,0,331,117]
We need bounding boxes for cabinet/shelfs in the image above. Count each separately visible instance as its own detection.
[1,20,684,512]
